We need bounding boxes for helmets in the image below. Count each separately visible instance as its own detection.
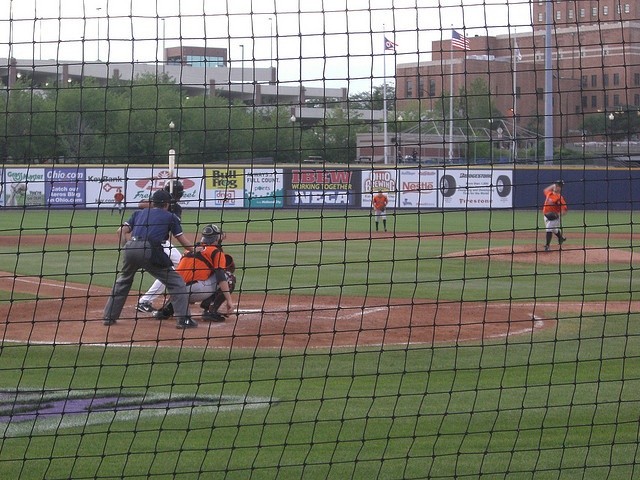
[554,178,566,185]
[163,178,184,202]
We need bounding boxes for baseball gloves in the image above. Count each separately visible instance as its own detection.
[380,206,386,212]
[545,211,559,220]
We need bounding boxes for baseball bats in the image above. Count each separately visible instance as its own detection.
[167,148,175,211]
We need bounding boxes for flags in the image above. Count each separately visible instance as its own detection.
[515,43,522,61]
[453,30,472,51]
[384,36,399,50]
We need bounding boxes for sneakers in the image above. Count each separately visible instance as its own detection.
[151,309,170,320]
[175,318,198,329]
[544,244,550,252]
[134,303,157,313]
[558,235,566,245]
[102,316,116,325]
[201,309,225,322]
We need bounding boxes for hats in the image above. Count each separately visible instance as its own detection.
[152,188,172,203]
[200,223,224,236]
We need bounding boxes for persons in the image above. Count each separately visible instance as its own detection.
[135,180,184,314]
[102,189,203,328]
[543,180,567,251]
[152,223,235,321]
[111,190,124,215]
[373,190,388,233]
